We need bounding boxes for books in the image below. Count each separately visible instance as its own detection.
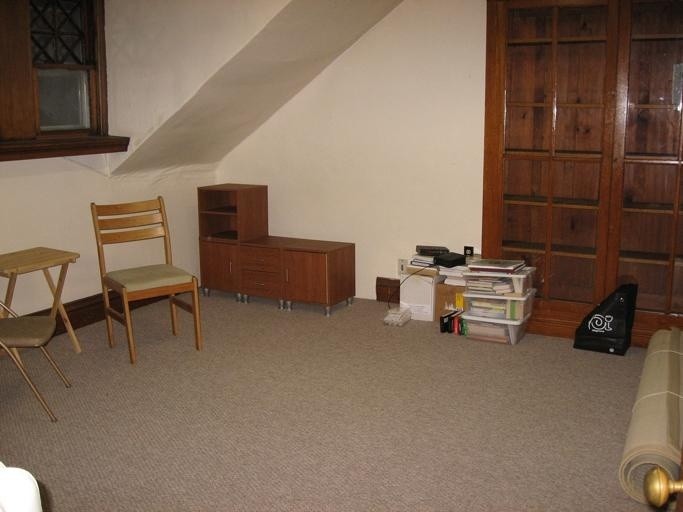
[392,245,538,344]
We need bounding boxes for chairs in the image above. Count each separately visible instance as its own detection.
[88,196,203,365]
[0,299,74,422]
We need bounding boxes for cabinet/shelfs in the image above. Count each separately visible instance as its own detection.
[240,235,285,311]
[284,237,356,317]
[196,183,269,243]
[198,234,241,304]
[481,0,683,341]
